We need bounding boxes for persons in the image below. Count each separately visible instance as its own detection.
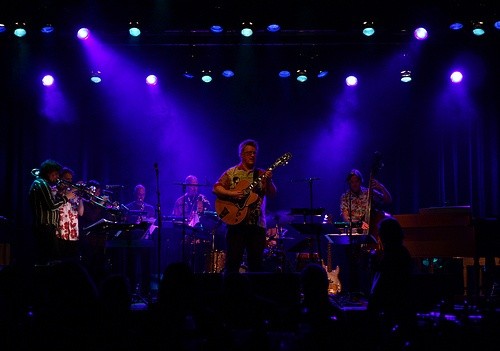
[28,159,62,281]
[77,180,118,269]
[49,167,88,274]
[211,140,277,272]
[121,184,156,295]
[339,168,390,232]
[172,175,211,243]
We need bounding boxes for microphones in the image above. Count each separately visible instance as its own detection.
[153,162,159,176]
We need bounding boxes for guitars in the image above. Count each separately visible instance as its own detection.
[214,151,293,224]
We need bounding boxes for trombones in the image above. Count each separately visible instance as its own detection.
[31,168,113,209]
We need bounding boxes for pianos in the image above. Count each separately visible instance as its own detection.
[370,206,500,297]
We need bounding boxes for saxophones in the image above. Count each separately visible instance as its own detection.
[137,199,144,223]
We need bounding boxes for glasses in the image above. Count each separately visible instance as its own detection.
[243,151,256,154]
